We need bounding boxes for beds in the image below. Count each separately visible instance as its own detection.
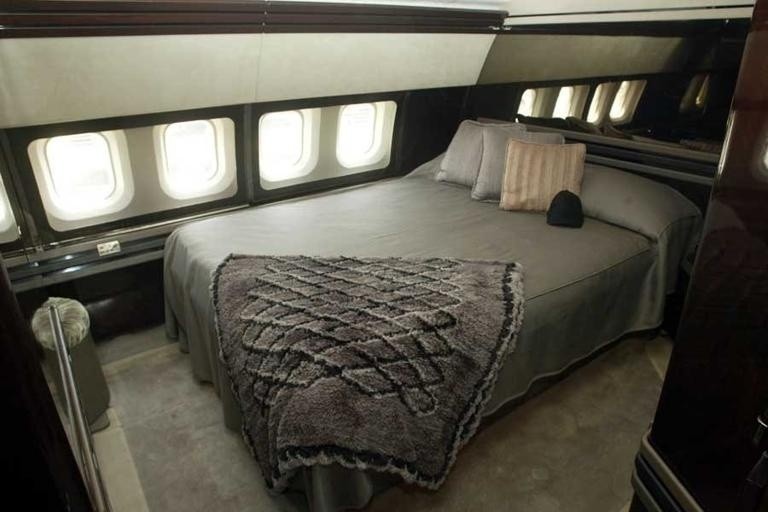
[161,151,704,510]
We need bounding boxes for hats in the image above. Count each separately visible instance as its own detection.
[546,190,583,228]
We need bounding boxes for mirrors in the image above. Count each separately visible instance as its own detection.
[467,3,755,179]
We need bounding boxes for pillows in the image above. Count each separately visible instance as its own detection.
[498,138,589,213]
[470,125,567,202]
[436,118,527,188]
